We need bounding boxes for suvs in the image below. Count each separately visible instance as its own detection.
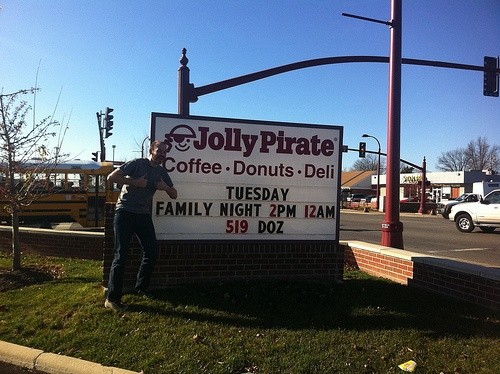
[436,193,480,219]
[449,188,500,233]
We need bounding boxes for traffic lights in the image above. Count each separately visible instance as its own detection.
[106,127,113,138]
[105,107,113,129]
[359,142,365,158]
[91,151,98,162]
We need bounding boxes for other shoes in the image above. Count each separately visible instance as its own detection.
[138,288,153,300]
[105,299,122,312]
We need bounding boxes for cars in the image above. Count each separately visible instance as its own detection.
[347,194,365,202]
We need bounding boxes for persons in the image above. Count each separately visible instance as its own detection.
[105,140,177,312]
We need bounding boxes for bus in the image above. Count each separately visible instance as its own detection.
[0,162,121,227]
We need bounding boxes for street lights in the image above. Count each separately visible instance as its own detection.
[362,134,381,208]
